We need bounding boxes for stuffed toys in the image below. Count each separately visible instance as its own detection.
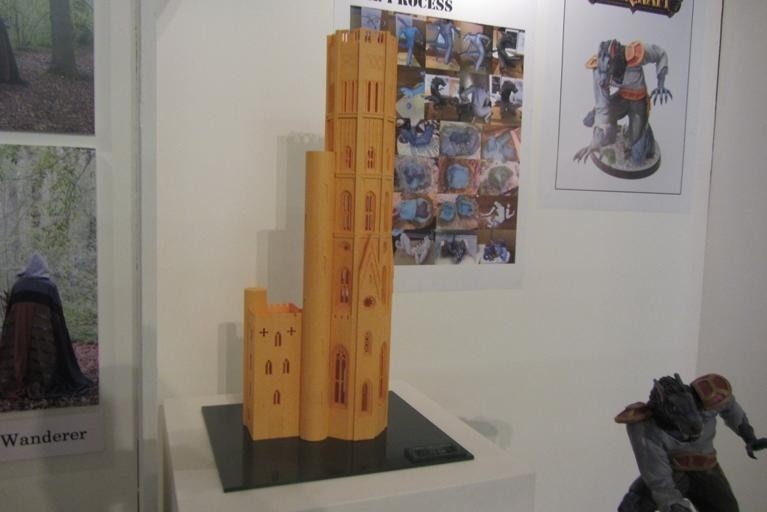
[614,371,767,511]
[572,37,675,178]
